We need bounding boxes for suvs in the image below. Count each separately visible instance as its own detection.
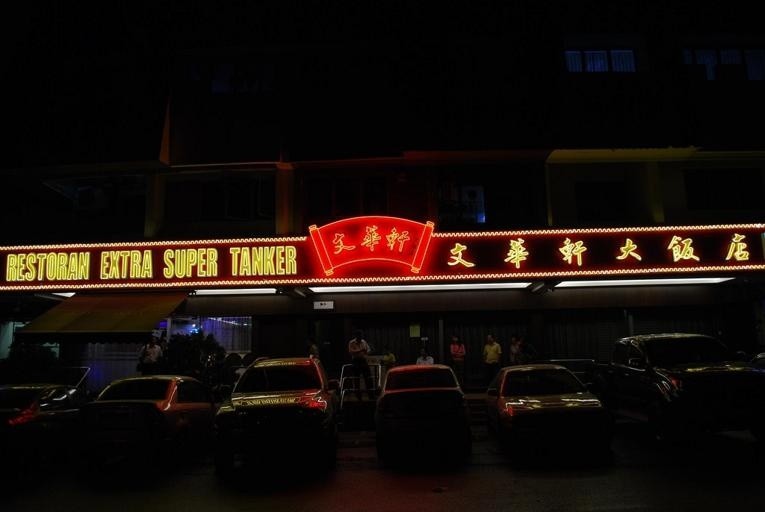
[585,332,765,439]
[213,353,341,465]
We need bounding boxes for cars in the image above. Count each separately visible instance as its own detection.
[486,363,602,452]
[78,374,221,451]
[0,383,95,460]
[374,362,469,448]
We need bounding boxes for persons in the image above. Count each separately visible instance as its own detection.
[483,335,536,387]
[450,335,465,385]
[380,348,396,368]
[349,331,375,400]
[416,346,434,366]
[139,336,163,364]
